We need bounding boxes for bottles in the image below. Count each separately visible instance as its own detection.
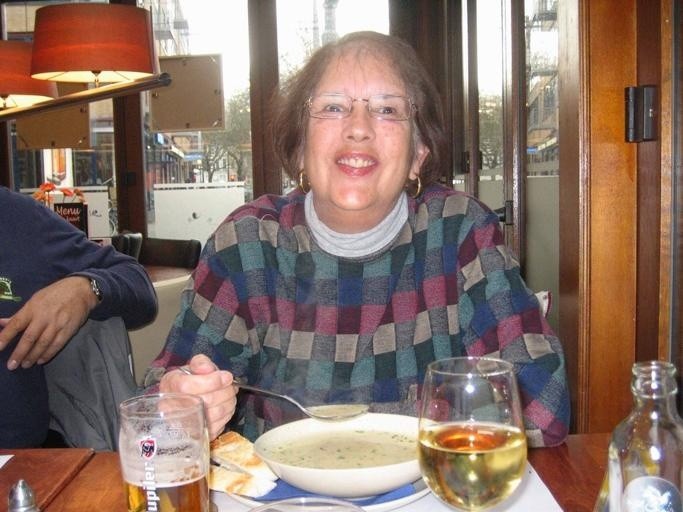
[608,361,683,512]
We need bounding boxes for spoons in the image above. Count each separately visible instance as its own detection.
[177,366,370,422]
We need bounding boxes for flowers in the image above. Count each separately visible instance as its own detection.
[32,181,85,218]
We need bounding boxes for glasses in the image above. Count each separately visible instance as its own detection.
[304,92,418,126]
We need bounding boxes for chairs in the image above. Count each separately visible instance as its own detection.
[125,275,194,391]
[140,237,201,268]
[117,232,143,263]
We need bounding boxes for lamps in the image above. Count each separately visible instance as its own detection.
[30,4,161,89]
[0,39,58,109]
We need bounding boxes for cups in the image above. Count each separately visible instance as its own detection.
[118,391,211,511]
[416,357,528,512]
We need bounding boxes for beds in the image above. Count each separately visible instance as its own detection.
[136,264,193,286]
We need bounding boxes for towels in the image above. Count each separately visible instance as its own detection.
[252,479,416,505]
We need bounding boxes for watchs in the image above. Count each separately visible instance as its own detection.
[82,274,105,312]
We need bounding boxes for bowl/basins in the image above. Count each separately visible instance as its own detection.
[252,412,438,501]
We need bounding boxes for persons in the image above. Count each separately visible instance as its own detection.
[134,30,573,451]
[0,182,161,450]
